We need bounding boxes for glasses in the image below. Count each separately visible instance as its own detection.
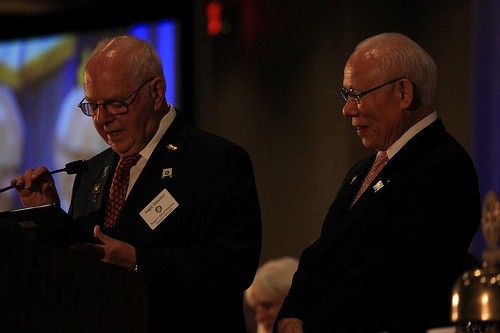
[78,78,151,116]
[341,76,403,104]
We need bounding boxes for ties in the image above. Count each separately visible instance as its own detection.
[349,150,390,209]
[104,153,143,229]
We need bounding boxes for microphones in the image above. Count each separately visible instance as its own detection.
[0,160,90,193]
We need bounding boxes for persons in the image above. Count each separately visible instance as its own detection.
[0,82,109,214]
[272,33,480,333]
[246,257,298,333]
[10,37,263,333]
